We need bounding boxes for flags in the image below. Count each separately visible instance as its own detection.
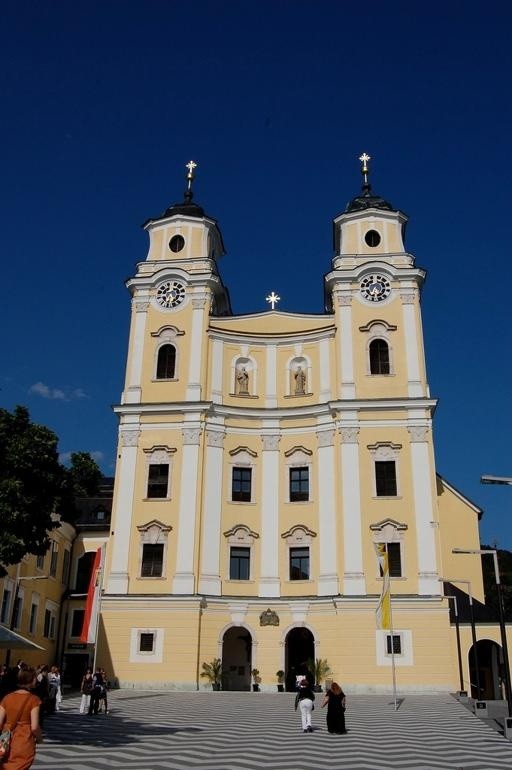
[79,542,106,644]
[373,542,393,630]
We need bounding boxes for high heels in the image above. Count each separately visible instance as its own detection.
[304,728,313,733]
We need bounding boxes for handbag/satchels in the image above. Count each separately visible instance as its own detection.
[341,697,346,708]
[0,729,10,762]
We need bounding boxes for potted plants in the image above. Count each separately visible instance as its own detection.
[305,658,333,693]
[276,670,285,692]
[251,669,262,692]
[200,658,233,691]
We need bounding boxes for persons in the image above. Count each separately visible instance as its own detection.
[294,679,317,734]
[319,681,346,734]
[0,659,112,770]
[237,367,250,393]
[294,364,305,393]
